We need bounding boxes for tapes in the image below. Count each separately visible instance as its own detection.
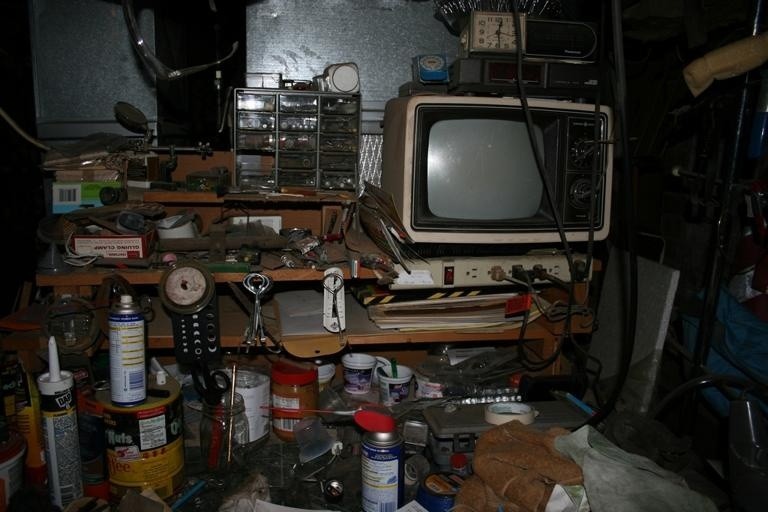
[485,401,540,426]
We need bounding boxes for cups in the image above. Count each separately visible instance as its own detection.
[377,365,413,408]
[341,354,376,394]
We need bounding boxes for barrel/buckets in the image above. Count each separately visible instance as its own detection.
[92,370,187,490]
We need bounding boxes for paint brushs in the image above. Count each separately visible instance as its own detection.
[259,405,358,416]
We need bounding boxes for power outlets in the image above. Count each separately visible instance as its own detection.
[385,255,594,292]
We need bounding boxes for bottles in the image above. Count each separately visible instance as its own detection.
[199,392,249,476]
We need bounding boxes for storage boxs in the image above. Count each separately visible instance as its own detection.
[422,400,587,475]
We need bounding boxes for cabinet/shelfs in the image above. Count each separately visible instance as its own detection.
[234,87,361,194]
[42,144,360,256]
[0,255,601,393]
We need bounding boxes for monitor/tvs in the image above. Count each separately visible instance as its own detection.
[378,92,614,248]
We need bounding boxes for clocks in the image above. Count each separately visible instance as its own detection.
[469,8,526,56]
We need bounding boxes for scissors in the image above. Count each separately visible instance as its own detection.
[227,274,285,354]
[189,366,231,407]
